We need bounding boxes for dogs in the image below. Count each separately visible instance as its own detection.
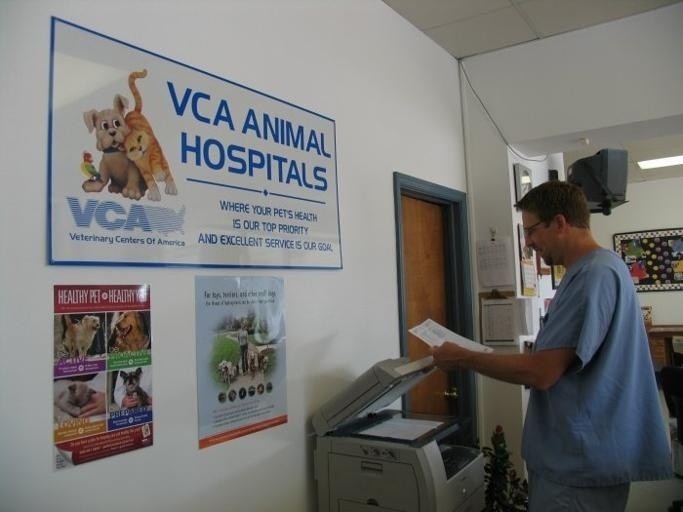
[60,313,101,363]
[82,92,148,200]
[118,367,150,409]
[113,311,146,352]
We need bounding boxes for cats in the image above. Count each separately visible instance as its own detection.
[57,381,98,418]
[119,66,179,202]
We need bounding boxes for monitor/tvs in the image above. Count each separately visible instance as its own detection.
[567,147,630,215]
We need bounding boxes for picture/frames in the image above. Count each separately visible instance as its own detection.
[613,229,683,293]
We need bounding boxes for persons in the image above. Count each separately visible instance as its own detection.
[237,320,249,376]
[78,373,106,417]
[428,180,672,512]
[114,366,152,418]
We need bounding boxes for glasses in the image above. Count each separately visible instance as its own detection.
[524,219,544,236]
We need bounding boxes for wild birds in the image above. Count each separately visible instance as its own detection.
[78,149,104,181]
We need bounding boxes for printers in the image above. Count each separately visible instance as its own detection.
[310,352,487,510]
